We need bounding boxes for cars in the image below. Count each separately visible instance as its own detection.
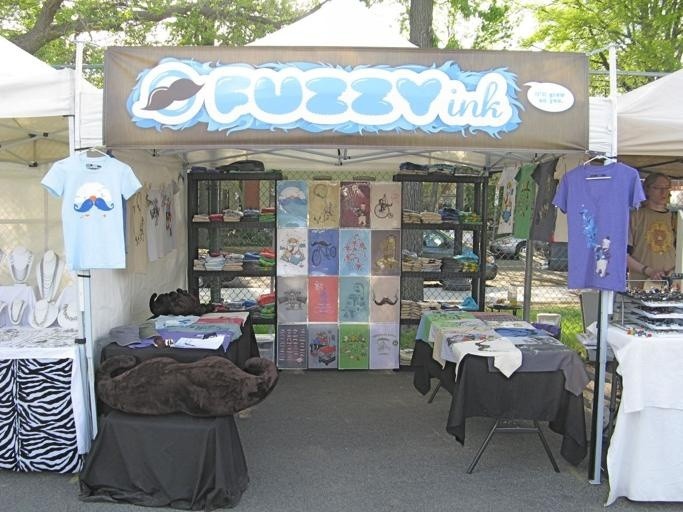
[423,228,498,280]
[488,234,538,262]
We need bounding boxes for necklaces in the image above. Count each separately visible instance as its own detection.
[32,304,48,326]
[0,326,80,348]
[40,254,58,304]
[9,251,31,282]
[62,304,79,321]
[9,300,24,324]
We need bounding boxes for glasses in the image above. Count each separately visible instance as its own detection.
[648,186,672,192]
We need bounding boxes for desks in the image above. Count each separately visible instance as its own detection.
[103,309,251,372]
[423,308,578,476]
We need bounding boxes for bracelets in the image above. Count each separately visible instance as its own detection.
[641,265,648,277]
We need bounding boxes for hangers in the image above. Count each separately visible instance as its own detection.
[58,140,130,180]
[563,153,639,182]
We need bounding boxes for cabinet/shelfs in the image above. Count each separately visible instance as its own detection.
[399,176,486,371]
[187,171,276,371]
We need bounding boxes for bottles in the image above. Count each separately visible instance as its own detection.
[508,283,517,306]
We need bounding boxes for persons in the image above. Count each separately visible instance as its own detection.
[624,172,682,295]
